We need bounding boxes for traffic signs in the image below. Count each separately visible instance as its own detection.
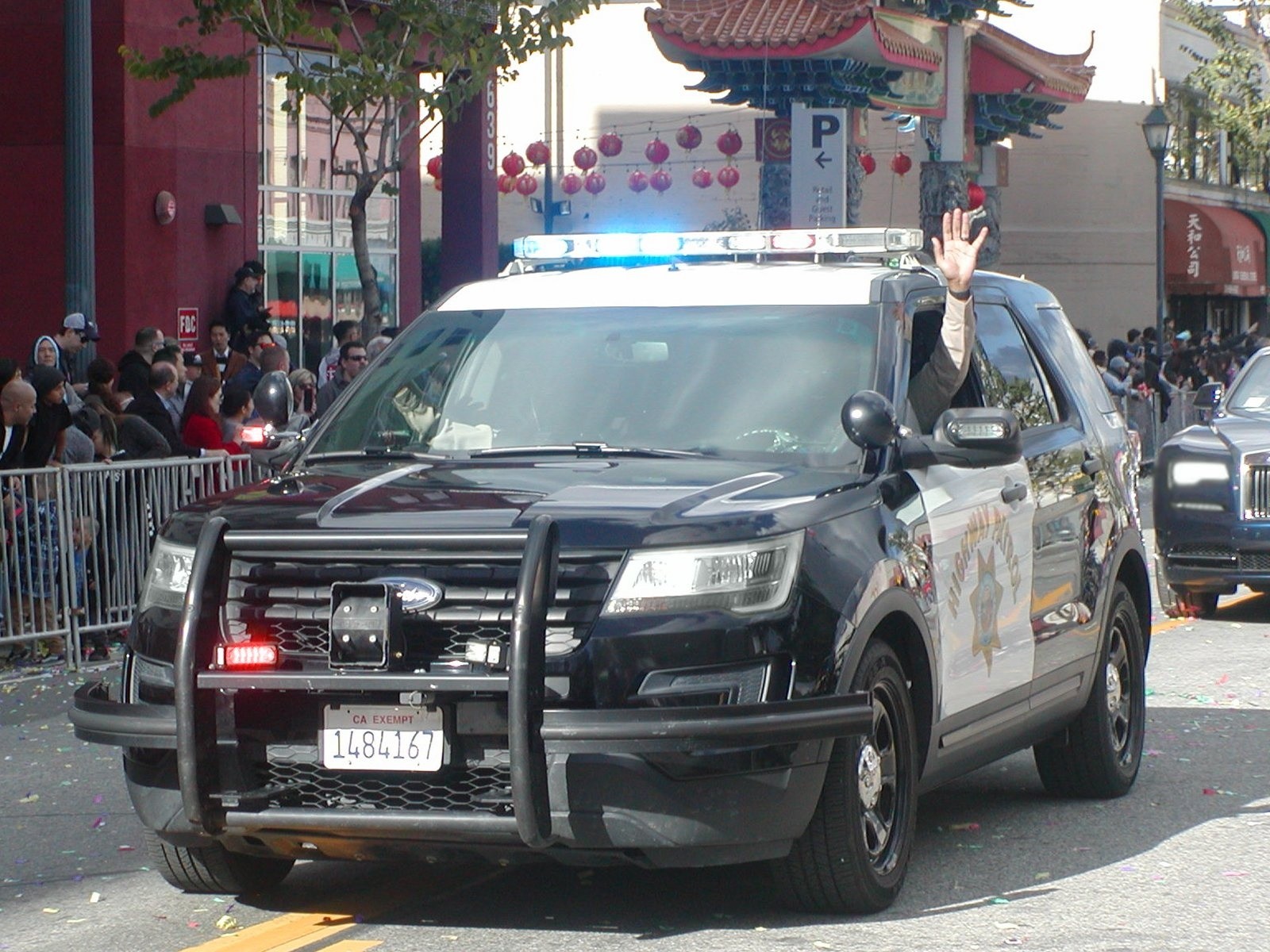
[790,102,848,229]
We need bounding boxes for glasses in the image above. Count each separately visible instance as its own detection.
[348,355,367,362]
[77,331,89,342]
[153,341,165,346]
[300,382,316,390]
[255,343,276,349]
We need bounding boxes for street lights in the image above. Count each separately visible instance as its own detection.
[1138,96,1172,361]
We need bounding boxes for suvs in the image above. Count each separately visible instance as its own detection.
[1152,347,1270,618]
[67,229,1153,914]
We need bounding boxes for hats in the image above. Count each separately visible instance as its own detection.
[1109,356,1130,369]
[235,266,260,280]
[244,260,265,275]
[33,365,66,396]
[183,352,204,365]
[64,313,101,343]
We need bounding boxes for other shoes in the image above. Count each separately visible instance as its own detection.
[0,629,131,670]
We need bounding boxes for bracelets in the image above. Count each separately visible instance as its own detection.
[948,284,973,300]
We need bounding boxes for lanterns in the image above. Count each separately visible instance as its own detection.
[644,138,669,164]
[692,168,714,188]
[675,125,702,153]
[966,177,986,210]
[859,153,876,175]
[628,171,648,193]
[497,133,622,202]
[890,151,912,179]
[427,157,442,190]
[650,169,673,194]
[717,165,740,192]
[717,129,742,161]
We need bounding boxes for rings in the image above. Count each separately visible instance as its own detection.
[961,237,970,241]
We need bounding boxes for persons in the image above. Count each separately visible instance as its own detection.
[0,260,401,665]
[1082,317,1270,480]
[905,208,989,433]
[392,381,557,450]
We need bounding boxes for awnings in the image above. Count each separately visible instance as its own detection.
[1164,201,1270,306]
[267,252,390,302]
